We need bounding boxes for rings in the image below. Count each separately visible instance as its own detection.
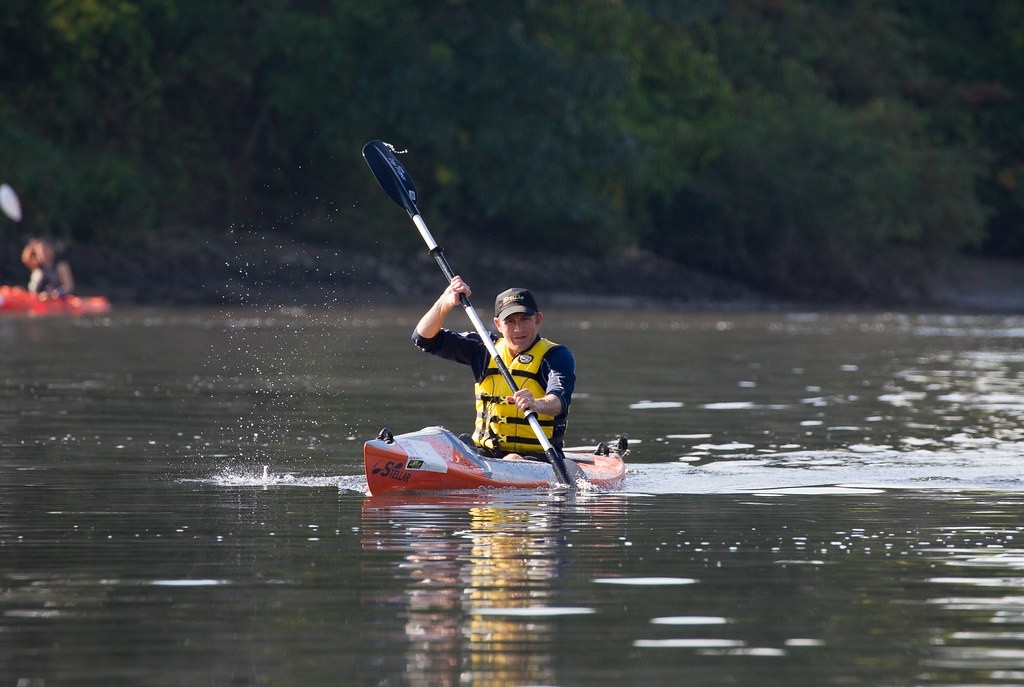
[524,396,529,400]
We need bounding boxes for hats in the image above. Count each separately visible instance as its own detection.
[495,287,539,320]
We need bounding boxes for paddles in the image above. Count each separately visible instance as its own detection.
[0,183,67,302]
[362,139,587,490]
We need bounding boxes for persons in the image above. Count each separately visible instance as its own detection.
[21,238,73,304]
[411,276,576,464]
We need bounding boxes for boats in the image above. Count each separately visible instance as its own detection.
[362,425,631,496]
[0,284,113,319]
